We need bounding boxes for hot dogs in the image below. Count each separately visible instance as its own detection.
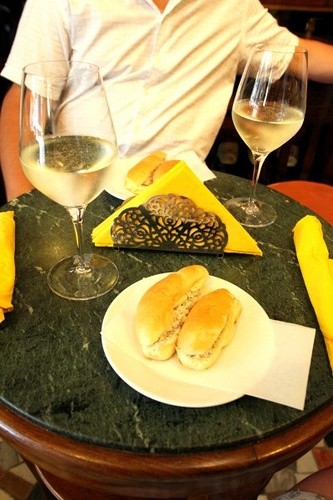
[134,264,209,362]
[175,289,242,371]
[126,148,180,190]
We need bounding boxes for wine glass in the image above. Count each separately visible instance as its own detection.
[18,61,120,302]
[222,43,309,228]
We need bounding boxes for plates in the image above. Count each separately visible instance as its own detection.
[100,272,277,410]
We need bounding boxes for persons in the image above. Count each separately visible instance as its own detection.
[0,1,332,203]
